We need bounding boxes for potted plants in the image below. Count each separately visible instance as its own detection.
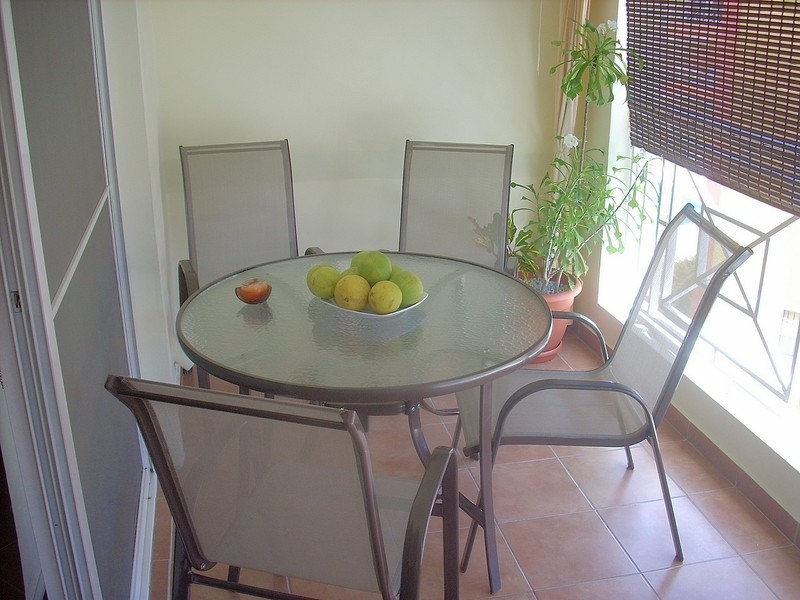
[468,17,666,364]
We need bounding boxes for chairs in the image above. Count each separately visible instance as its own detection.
[105,139,754,600]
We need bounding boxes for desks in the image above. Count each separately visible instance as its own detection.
[176,251,553,595]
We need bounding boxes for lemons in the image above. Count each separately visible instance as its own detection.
[307,251,422,315]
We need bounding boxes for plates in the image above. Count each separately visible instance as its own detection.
[321,289,429,318]
